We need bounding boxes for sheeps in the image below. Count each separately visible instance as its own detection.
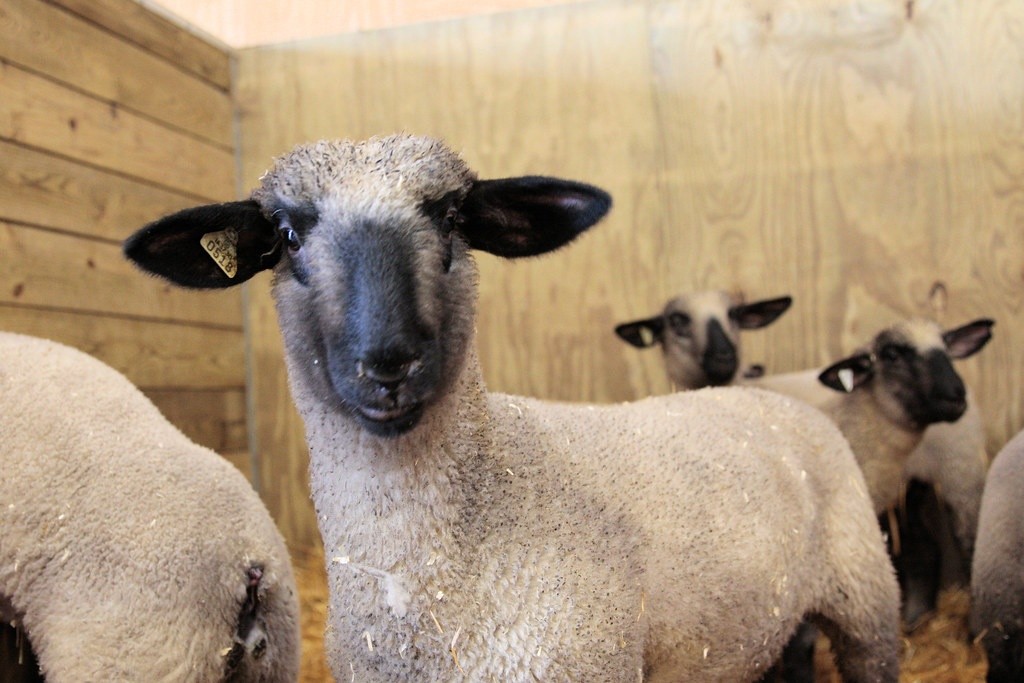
[612,288,1024,683]
[125,137,905,683]
[0,335,301,683]
